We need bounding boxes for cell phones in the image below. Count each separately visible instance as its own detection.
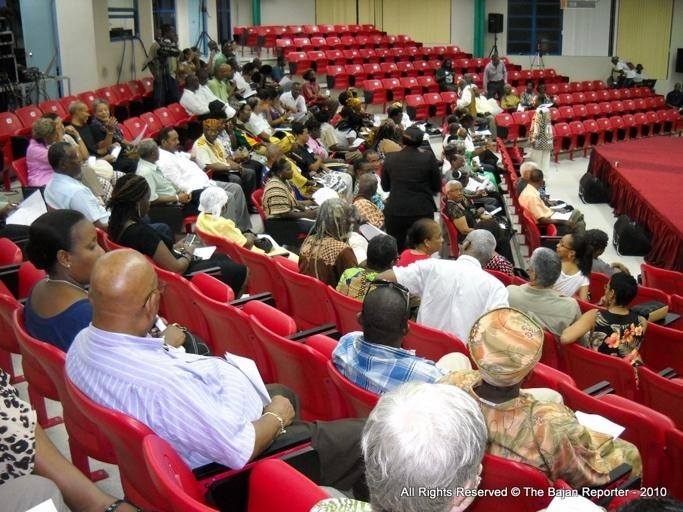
[185,233,196,246]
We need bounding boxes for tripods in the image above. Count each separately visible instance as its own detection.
[530,39,545,69]
[488,34,498,58]
[195,0,213,56]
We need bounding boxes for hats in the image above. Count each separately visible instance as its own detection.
[202,118,220,128]
[465,304,548,389]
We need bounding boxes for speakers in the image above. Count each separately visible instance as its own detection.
[676,48,683,74]
[488,13,503,33]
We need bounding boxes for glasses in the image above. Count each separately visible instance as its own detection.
[139,279,169,308]
[558,239,574,251]
[361,279,411,320]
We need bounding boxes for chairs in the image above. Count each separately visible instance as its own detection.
[0,22,682,512]
